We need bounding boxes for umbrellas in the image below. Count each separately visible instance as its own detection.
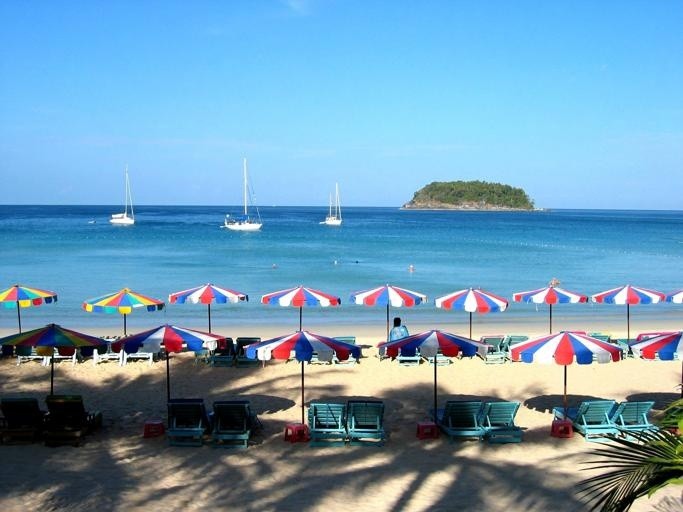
[591,283,665,339]
[634,331,682,394]
[108,323,225,407]
[512,285,586,333]
[166,281,250,334]
[504,331,624,419]
[349,283,427,344]
[0,283,58,333]
[81,286,165,339]
[260,285,342,330]
[372,329,489,429]
[663,286,683,306]
[0,324,123,396]
[246,332,360,424]
[436,285,506,338]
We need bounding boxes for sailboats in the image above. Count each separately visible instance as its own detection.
[224,156,262,232]
[109,168,134,225]
[323,182,343,227]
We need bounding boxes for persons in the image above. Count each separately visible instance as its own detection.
[389,317,409,342]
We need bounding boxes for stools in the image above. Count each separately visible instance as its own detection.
[144,420,161,439]
[417,423,437,440]
[283,424,306,443]
[551,421,571,438]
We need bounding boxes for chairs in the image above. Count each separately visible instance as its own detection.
[552,401,617,443]
[44,394,103,443]
[307,402,346,447]
[167,397,212,447]
[214,400,257,448]
[1,397,48,447]
[479,402,523,443]
[432,401,483,442]
[610,403,660,443]
[348,401,383,446]
